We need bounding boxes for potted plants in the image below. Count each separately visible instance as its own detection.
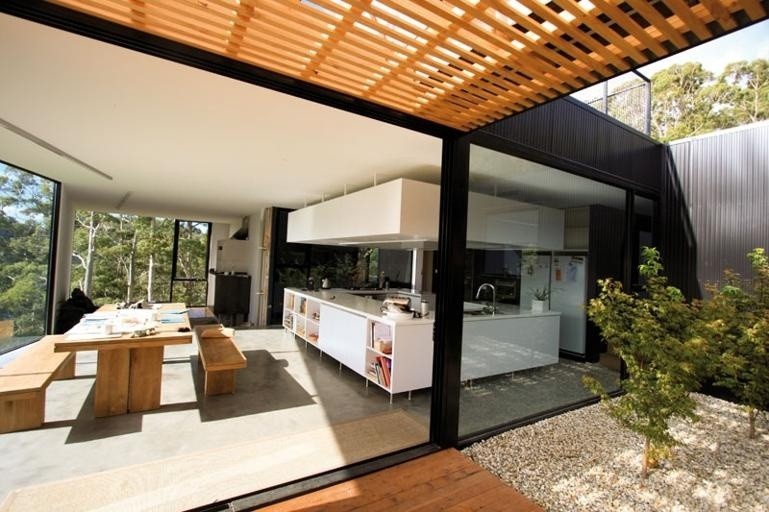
[530,286,559,312]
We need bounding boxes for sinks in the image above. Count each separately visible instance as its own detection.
[464,309,505,316]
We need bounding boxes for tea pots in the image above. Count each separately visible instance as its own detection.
[321,278,331,289]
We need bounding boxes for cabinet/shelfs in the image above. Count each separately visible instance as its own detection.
[364,318,393,394]
[282,289,321,349]
[214,273,251,325]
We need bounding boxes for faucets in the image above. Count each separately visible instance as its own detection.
[476,283,496,317]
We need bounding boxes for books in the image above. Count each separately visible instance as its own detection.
[370,357,390,387]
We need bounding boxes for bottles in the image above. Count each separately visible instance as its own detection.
[307,278,314,290]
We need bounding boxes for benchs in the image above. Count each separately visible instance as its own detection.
[194,323,247,397]
[0,334,75,433]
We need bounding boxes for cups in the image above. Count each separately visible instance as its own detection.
[150,313,157,321]
[104,324,113,334]
[421,300,429,315]
